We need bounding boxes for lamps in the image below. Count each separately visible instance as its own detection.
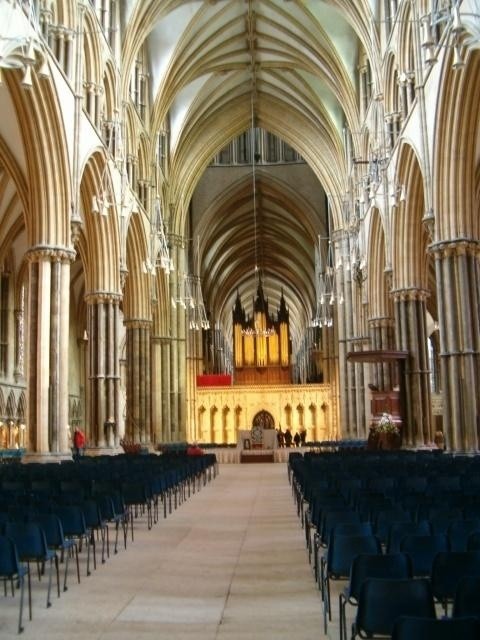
[0,0,210,333]
[309,1,480,328]
[239,92,275,337]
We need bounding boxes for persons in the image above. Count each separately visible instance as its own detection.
[277,429,306,448]
[73,426,85,457]
[186,442,203,455]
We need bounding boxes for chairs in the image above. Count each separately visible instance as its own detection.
[286,448,480,640]
[0,453,220,634]
